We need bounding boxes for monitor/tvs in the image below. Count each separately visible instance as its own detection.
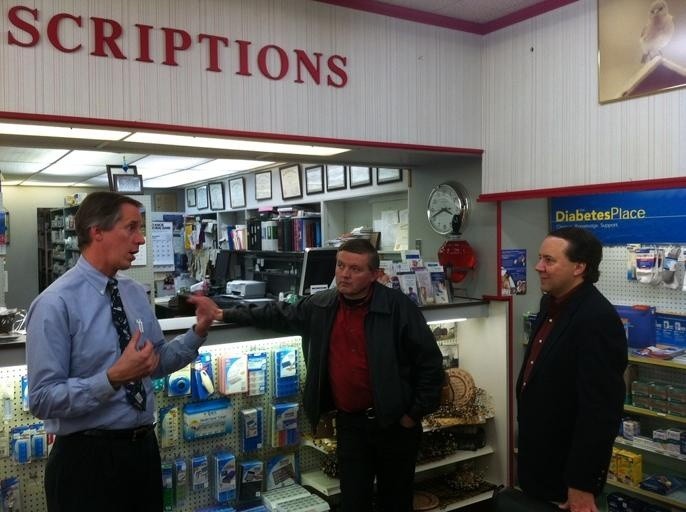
[298,247,340,297]
[213,252,233,285]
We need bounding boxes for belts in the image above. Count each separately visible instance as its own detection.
[84,423,157,444]
[340,406,375,422]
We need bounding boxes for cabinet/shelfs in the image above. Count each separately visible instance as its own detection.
[37,205,81,295]
[301,411,497,512]
[605,346,686,512]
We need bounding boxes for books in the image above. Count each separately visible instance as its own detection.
[374,248,449,309]
[221,213,321,252]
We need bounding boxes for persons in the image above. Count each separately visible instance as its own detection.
[184,240,446,510]
[514,228,630,512]
[26,192,216,512]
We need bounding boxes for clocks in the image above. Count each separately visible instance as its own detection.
[426,180,470,237]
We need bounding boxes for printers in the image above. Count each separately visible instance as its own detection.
[222,279,267,298]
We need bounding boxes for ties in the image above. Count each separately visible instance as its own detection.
[108,278,147,411]
